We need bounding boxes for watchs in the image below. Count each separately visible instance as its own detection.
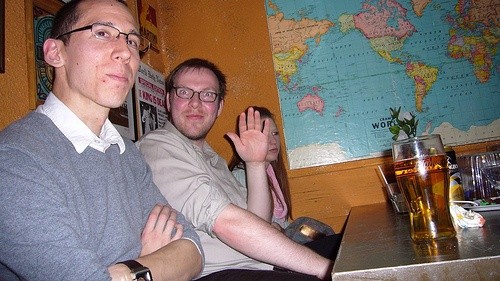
[116,260,153,281]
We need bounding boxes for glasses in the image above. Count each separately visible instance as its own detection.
[54,21,152,53]
[169,84,221,104]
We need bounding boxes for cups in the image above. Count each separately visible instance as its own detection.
[386,183,408,214]
[458,152,500,200]
[392,133,456,243]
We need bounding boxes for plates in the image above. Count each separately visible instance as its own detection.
[462,197,500,211]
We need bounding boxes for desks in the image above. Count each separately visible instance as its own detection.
[331,203,500,281]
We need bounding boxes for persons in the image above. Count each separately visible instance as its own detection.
[228,105,335,246]
[0,0,205,281]
[134,57,335,281]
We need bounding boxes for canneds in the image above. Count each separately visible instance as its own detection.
[429,145,463,209]
